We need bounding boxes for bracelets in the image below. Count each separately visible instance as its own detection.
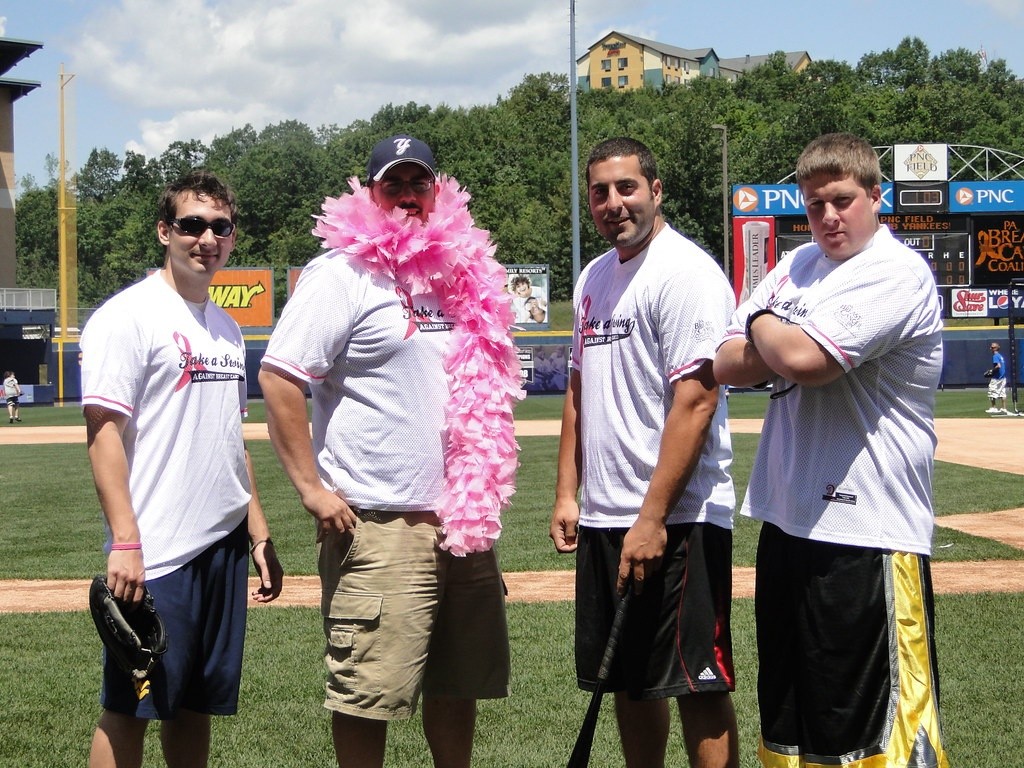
[111,543,142,550]
[250,539,273,555]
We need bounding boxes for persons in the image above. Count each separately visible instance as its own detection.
[76,174,285,768]
[510,276,548,323]
[2,371,22,423]
[257,134,512,768]
[710,130,954,768]
[546,137,742,768]
[984,342,1007,413]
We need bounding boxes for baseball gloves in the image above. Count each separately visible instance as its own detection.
[89,574,168,682]
[984,369,994,378]
[20,391,24,396]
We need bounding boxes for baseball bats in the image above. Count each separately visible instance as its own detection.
[566,570,634,768]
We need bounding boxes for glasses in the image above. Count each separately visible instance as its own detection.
[375,174,433,195]
[168,217,235,238]
[991,347,996,349]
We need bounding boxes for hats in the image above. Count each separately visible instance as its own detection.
[366,134,435,185]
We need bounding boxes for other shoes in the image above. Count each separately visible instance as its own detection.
[984,408,997,414]
[10,415,13,424]
[996,408,1007,415]
[15,415,21,420]
